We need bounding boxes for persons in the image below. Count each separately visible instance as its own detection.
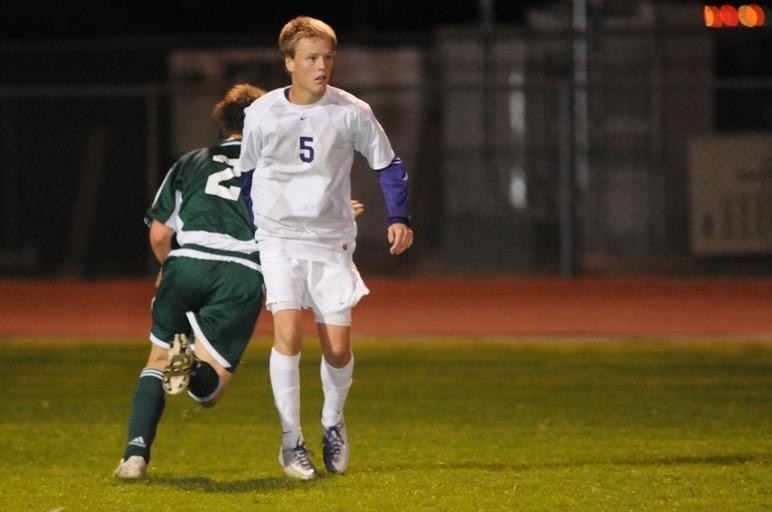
[110,83,363,481]
[239,17,416,481]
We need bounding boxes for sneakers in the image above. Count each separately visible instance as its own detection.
[162,331,194,395]
[278,444,319,480]
[113,454,146,478]
[320,412,349,474]
